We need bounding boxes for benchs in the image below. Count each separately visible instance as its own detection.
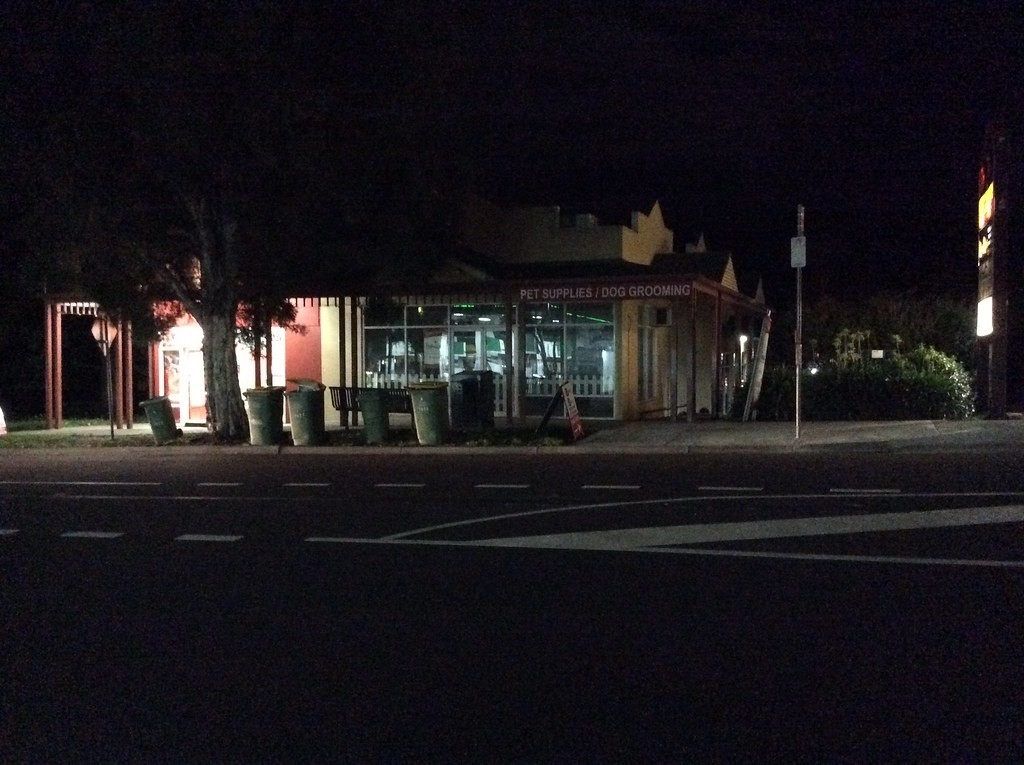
[328,386,415,429]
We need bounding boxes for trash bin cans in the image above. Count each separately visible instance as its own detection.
[283,377,327,446]
[404,381,452,448]
[357,389,390,447]
[243,384,287,446]
[138,393,184,446]
